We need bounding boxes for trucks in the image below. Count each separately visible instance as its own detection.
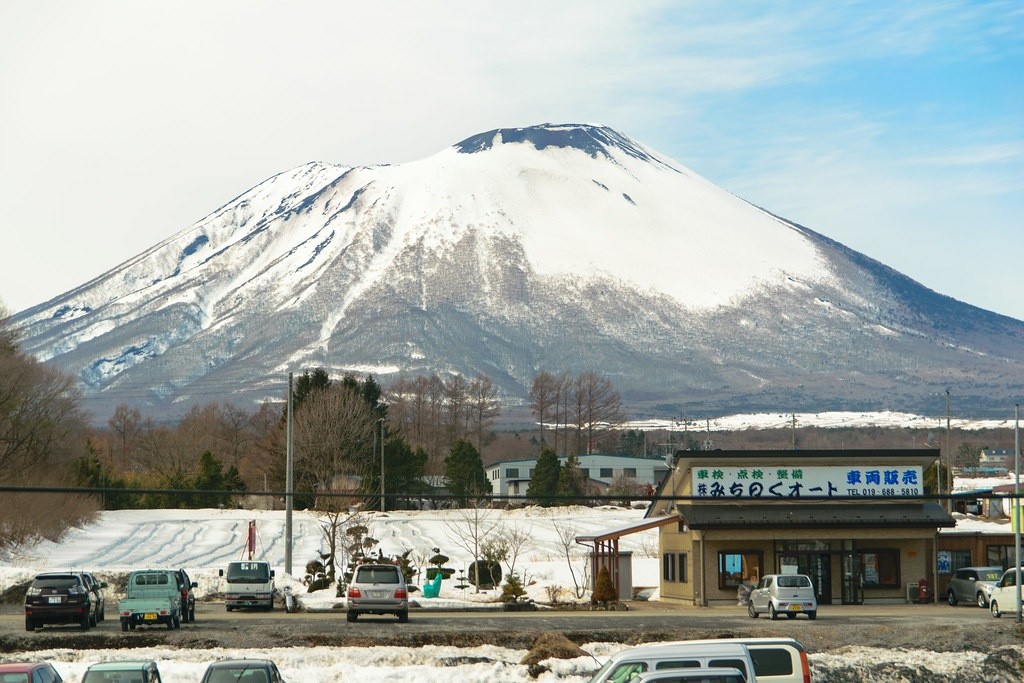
[218,560,275,612]
[118,568,182,631]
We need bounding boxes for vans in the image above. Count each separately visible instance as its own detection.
[588,643,758,683]
[629,667,748,683]
[641,637,811,683]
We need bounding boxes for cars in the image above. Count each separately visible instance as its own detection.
[25,571,109,631]
[81,660,162,683]
[200,658,286,683]
[989,567,1024,618]
[346,564,408,622]
[0,662,63,683]
[747,574,817,620]
[147,568,198,622]
[948,567,1004,608]
